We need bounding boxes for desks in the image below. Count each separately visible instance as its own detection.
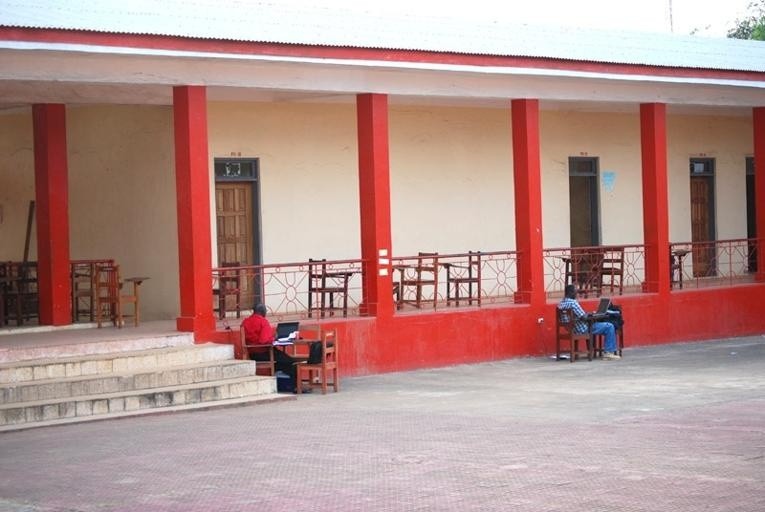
[590,314,624,358]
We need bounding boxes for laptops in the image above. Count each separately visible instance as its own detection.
[274,322,299,341]
[592,297,612,316]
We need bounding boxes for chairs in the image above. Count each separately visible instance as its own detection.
[212,262,242,320]
[391,250,482,310]
[560,246,624,299]
[308,258,352,317]
[240,324,339,394]
[555,306,597,363]
[97,265,151,329]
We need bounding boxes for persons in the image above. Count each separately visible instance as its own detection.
[241,303,310,393]
[558,285,621,360]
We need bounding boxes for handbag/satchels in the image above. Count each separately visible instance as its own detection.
[306,340,334,364]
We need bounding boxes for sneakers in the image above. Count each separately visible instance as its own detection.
[293,385,312,393]
[603,352,620,361]
[274,370,291,378]
